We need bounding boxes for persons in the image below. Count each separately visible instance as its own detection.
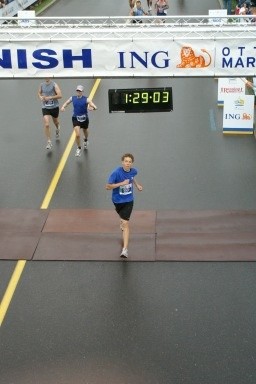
[235,0,255,22]
[129,0,169,23]
[0,0,42,24]
[60,85,97,156]
[105,153,143,258]
[38,79,61,149]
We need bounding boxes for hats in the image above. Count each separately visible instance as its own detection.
[76,86,83,91]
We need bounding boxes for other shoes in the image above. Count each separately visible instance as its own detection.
[77,147,82,153]
[56,129,60,137]
[46,144,52,149]
[84,141,88,149]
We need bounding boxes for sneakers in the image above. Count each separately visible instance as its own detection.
[120,248,128,258]
[120,219,123,231]
